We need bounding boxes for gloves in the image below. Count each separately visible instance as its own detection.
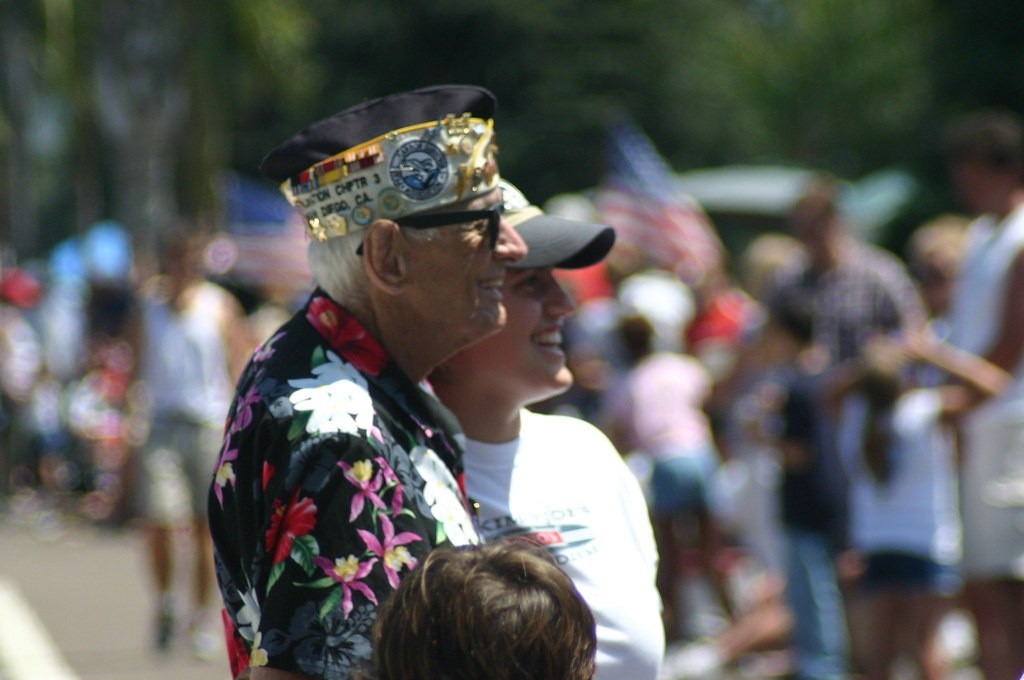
[892,387,943,439]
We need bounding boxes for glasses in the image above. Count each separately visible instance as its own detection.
[355,202,505,255]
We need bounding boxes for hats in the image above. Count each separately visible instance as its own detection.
[499,178,616,270]
[262,85,502,241]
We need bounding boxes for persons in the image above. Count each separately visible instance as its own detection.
[546,119,1022,678]
[429,178,665,679]
[1,223,254,649]
[208,84,525,680]
[368,536,604,679]
